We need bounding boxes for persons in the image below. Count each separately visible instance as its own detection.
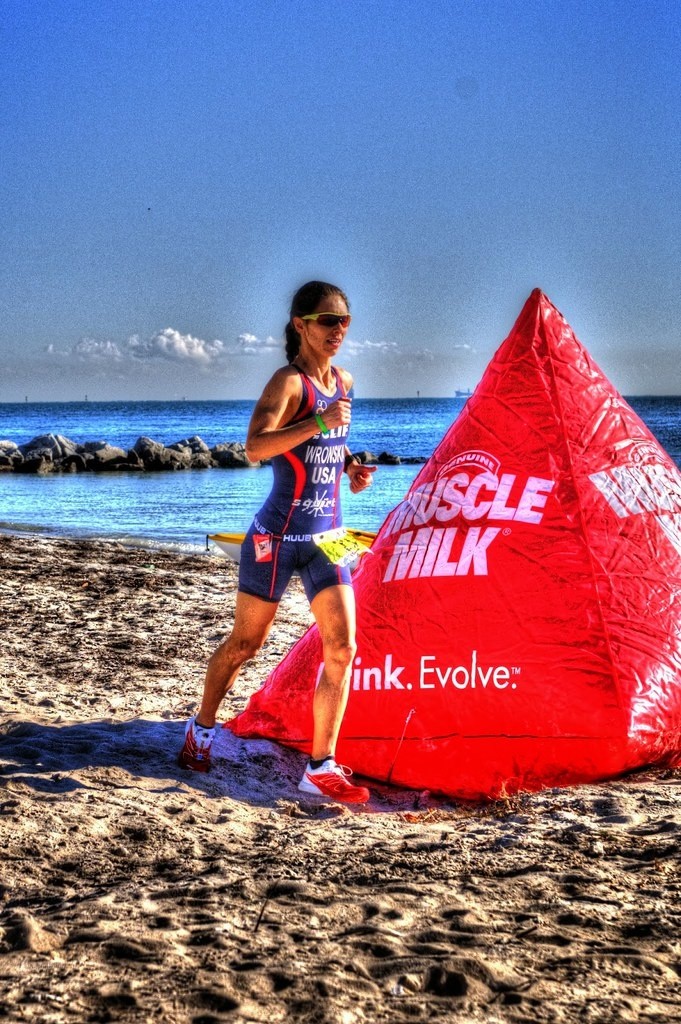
[179,282,378,802]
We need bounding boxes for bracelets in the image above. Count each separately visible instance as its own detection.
[316,414,329,434]
[343,454,361,473]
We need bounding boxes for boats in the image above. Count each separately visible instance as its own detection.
[206,528,378,564]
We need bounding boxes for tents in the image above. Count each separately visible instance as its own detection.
[221,288,681,800]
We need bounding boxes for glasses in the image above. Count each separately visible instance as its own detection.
[296,312,350,328]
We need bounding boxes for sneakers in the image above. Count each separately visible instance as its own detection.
[179,715,216,773]
[297,758,370,804]
